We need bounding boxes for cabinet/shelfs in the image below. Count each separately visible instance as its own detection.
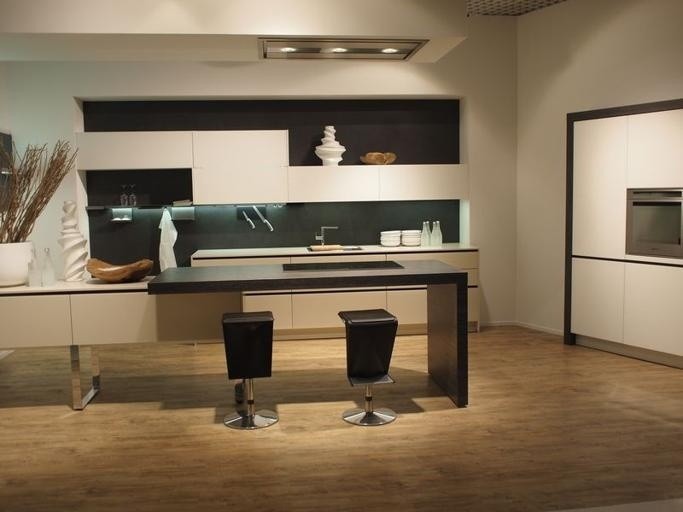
[0,275,243,350]
[190,251,479,330]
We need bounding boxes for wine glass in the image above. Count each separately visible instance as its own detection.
[119,183,136,206]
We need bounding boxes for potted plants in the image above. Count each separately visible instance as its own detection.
[0,135,81,287]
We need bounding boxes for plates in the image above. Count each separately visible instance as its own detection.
[380,229,421,247]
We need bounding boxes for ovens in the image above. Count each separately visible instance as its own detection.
[625,188,683,260]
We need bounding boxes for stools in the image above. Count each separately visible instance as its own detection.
[338,309,397,427]
[222,312,279,429]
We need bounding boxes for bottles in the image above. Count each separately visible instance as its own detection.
[29,245,55,288]
[421,220,444,247]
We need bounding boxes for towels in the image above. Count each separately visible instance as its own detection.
[158,208,178,272]
